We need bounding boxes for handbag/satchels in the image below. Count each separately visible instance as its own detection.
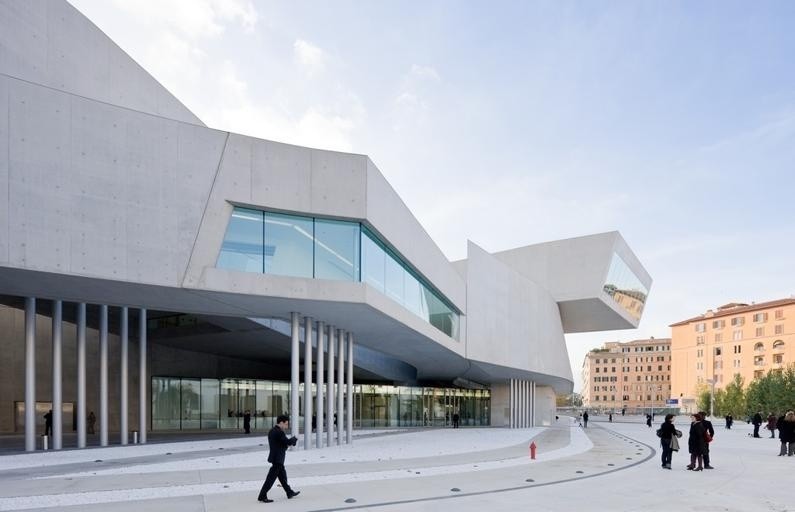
[657,429,663,437]
[705,432,712,442]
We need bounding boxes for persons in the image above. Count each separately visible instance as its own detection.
[226,408,338,435]
[257,414,301,503]
[401,408,472,429]
[86,411,96,434]
[40,409,52,437]
[583,411,589,428]
[607,407,795,471]
[578,413,583,428]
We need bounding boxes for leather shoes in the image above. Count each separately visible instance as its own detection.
[661,464,671,470]
[287,491,300,498]
[258,498,273,502]
[686,455,713,471]
